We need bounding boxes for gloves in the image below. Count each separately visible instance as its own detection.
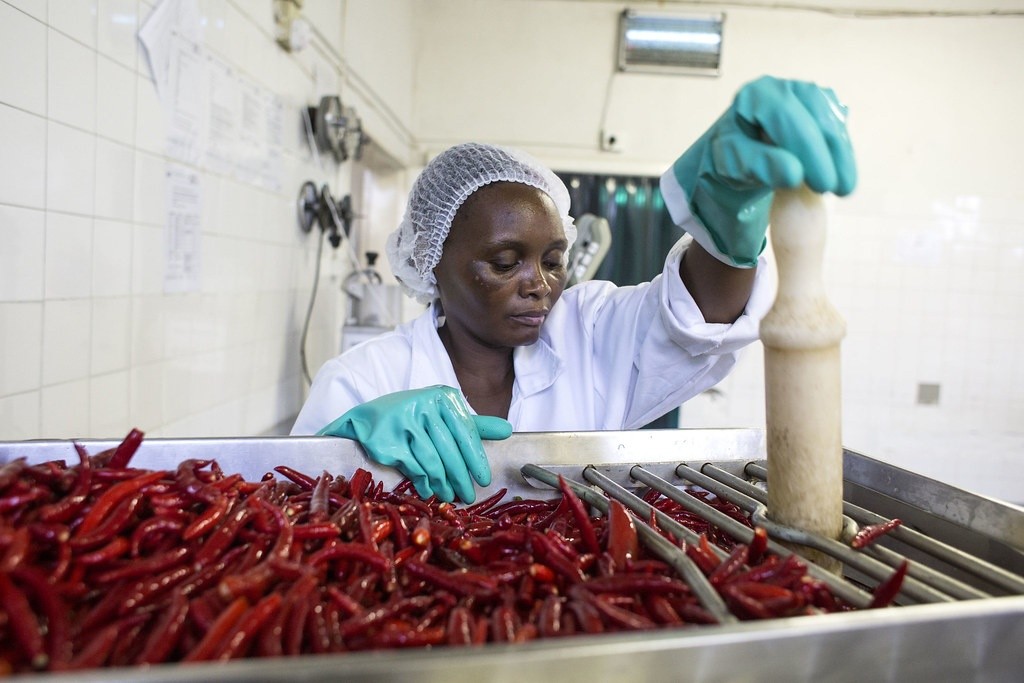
[315,384,513,505]
[658,75,858,268]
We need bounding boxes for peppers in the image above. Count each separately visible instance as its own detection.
[0,427,908,683]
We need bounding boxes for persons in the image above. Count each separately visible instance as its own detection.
[290,75,857,505]
